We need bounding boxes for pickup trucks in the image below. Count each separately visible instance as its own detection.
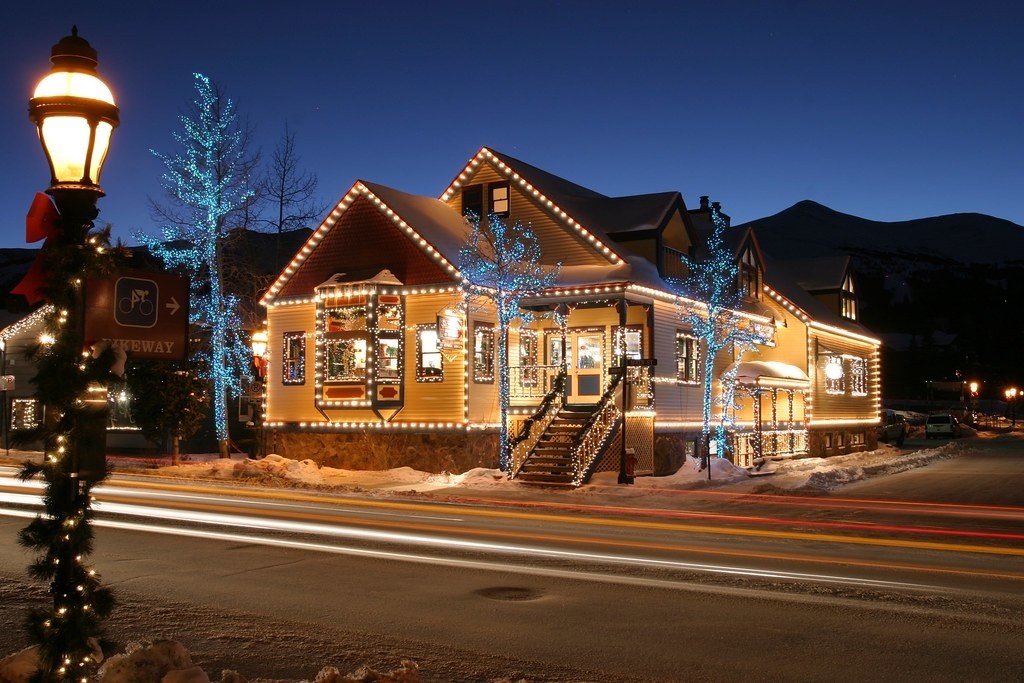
[878,409,910,447]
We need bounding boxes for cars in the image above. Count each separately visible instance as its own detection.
[925,414,961,439]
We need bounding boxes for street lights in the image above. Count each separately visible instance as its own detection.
[971,383,978,417]
[0,337,9,456]
[26,23,121,682]
[1006,388,1018,426]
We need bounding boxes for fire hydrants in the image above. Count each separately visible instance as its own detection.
[625,448,638,484]
